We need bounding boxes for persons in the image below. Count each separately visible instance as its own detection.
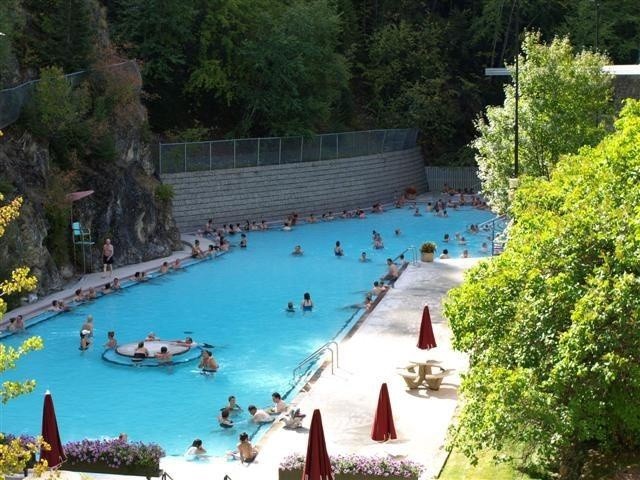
[102,431,130,449]
[184,391,306,466]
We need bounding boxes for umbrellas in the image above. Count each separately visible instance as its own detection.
[372,382,397,447]
[301,409,336,480]
[38,388,68,472]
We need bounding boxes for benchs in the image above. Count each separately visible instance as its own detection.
[395,353,456,392]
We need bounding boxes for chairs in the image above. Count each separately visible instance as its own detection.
[71,222,91,245]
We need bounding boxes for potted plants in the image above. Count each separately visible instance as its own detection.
[420,240,437,262]
[405,186,418,199]
